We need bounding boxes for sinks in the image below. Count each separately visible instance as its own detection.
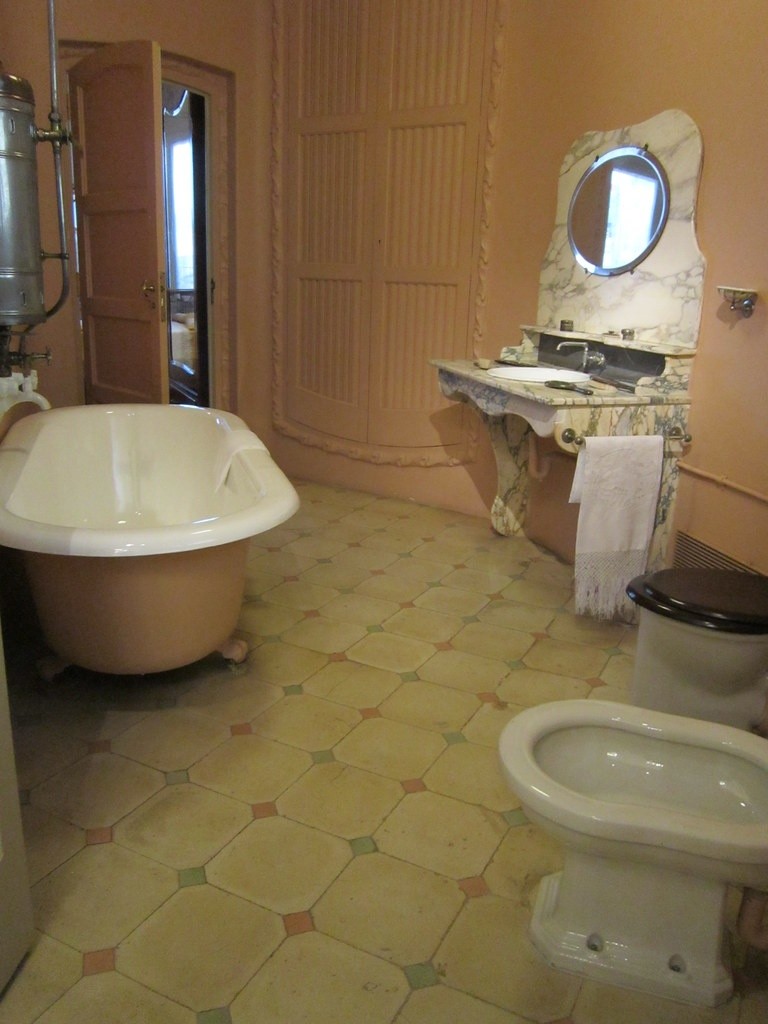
[485,366,590,384]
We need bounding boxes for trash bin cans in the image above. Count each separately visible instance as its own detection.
[623,568,767,736]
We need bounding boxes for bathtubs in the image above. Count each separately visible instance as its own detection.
[1,402,301,677]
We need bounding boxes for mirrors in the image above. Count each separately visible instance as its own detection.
[566,146,669,275]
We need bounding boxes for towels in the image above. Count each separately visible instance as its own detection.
[568,435,664,617]
[209,429,271,492]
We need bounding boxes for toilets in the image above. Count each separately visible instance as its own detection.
[495,695,767,1006]
[623,566,768,741]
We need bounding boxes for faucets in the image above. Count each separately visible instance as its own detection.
[1,386,51,413]
[555,341,589,372]
